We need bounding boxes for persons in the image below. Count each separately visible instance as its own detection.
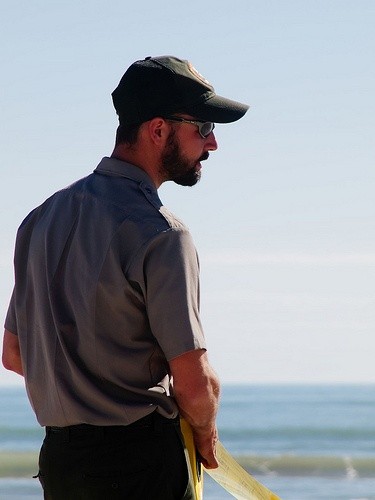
[2,56,250,500]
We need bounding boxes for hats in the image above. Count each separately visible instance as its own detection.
[111,56,249,123]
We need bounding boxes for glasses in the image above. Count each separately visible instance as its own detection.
[161,113,215,139]
